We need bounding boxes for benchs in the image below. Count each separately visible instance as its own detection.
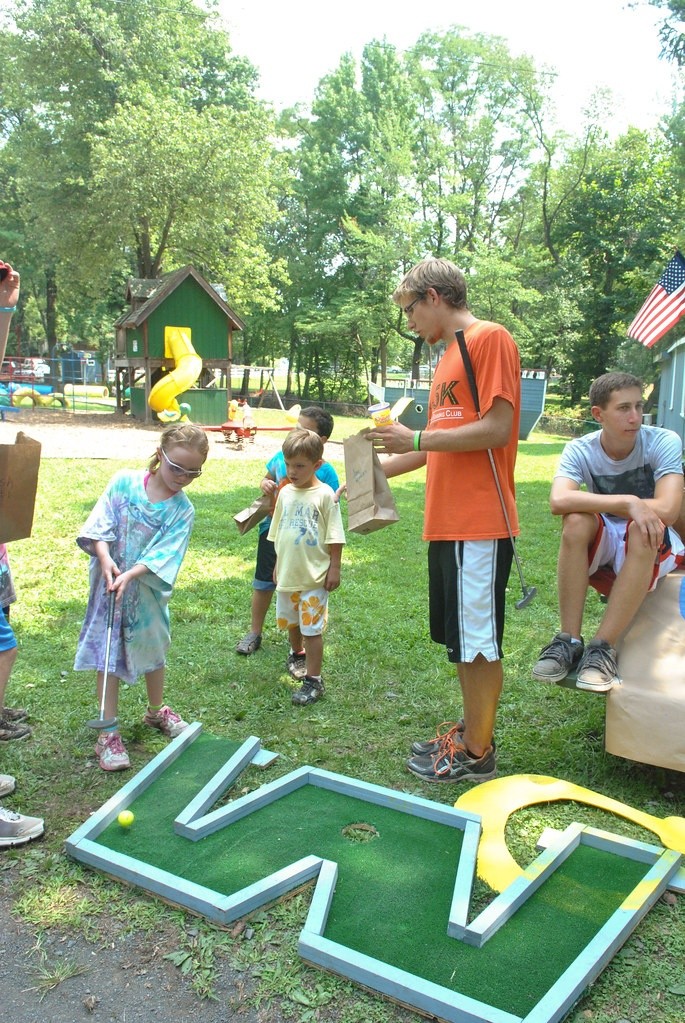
[604,566,685,775]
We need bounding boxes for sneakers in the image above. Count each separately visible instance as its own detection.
[236,633,262,653]
[292,675,325,706]
[144,706,189,737]
[0,707,31,744]
[286,649,308,680]
[95,733,130,770]
[576,639,621,692]
[0,774,16,798]
[407,719,497,782]
[532,632,585,682]
[0,807,45,846]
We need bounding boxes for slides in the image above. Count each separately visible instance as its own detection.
[146,326,203,423]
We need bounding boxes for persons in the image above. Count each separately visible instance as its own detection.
[73,421,209,771]
[334,255,521,783]
[532,372,685,692]
[0,260,43,847]
[235,405,346,707]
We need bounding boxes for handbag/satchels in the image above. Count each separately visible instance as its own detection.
[343,427,399,535]
[233,492,275,535]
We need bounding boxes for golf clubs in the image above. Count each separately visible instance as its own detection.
[455,328,538,611]
[86,572,119,730]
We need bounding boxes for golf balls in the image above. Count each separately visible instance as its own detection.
[117,810,136,828]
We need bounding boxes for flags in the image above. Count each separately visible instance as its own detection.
[625,252,685,349]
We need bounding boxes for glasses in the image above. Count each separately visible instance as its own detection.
[162,448,202,478]
[404,296,420,316]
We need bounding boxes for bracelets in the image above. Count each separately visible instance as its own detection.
[0,305,17,312]
[413,430,422,451]
